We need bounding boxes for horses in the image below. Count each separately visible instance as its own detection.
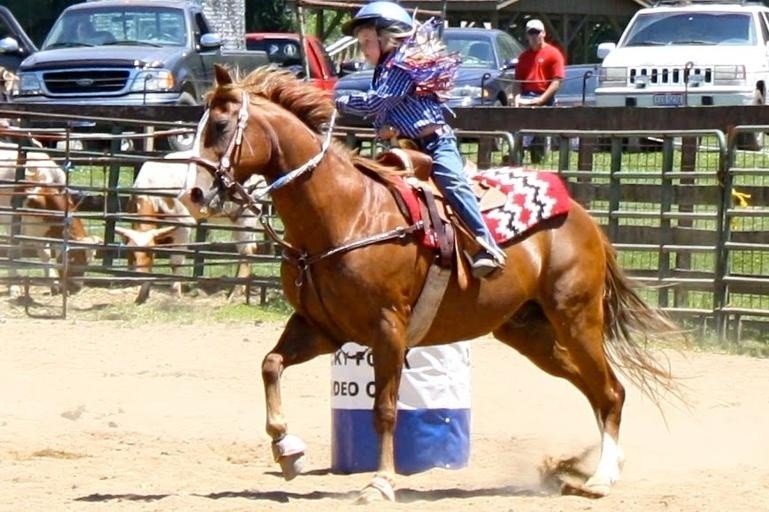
[188,61,696,506]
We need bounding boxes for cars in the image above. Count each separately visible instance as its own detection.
[515,60,603,151]
[247,26,337,92]
[339,24,527,129]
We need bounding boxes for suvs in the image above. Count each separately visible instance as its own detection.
[11,4,265,149]
[602,4,768,151]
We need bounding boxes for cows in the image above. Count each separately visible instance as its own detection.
[110,146,263,306]
[0,141,103,295]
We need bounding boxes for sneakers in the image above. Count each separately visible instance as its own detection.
[470,251,503,280]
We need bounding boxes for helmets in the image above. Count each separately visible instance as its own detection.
[342,1,412,35]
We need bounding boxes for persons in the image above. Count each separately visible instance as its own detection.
[68,17,102,46]
[332,1,507,282]
[504,17,567,167]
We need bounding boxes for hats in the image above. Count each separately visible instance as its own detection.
[526,19,544,32]
